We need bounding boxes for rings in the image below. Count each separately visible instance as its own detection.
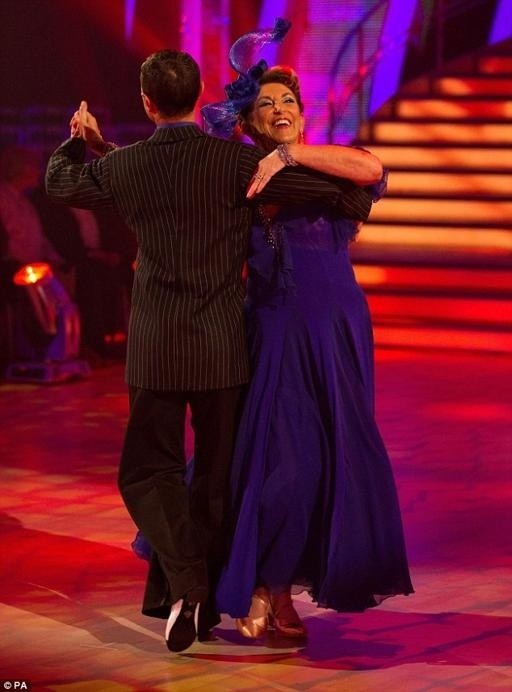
[256,175,263,181]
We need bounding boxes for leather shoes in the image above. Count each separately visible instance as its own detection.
[272,601,307,637]
[235,592,273,639]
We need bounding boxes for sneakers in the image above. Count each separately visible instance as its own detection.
[164,597,201,653]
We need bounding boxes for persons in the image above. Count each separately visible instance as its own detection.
[46,48,373,654]
[68,63,417,641]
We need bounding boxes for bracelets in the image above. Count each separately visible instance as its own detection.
[276,143,299,168]
